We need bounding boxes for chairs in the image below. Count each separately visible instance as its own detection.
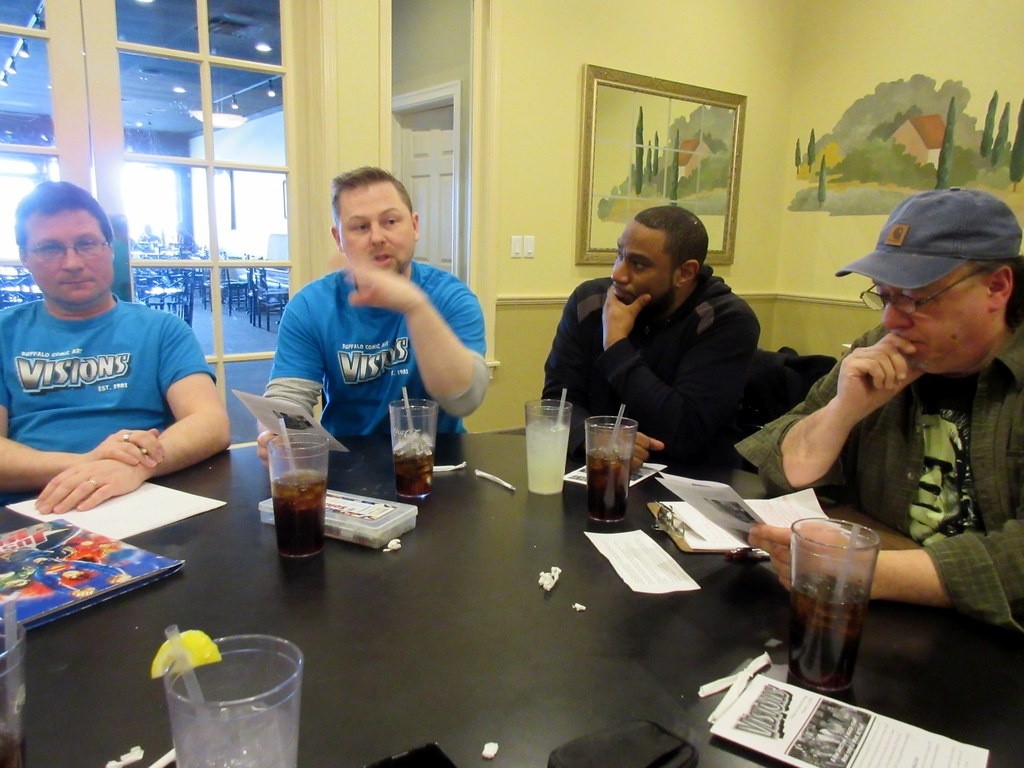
[131,245,288,332]
[755,349,837,423]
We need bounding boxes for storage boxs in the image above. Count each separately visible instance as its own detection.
[258,488,418,549]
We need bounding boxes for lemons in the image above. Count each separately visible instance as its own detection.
[151,629,222,678]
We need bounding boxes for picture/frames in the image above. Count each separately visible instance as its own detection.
[575,64,747,266]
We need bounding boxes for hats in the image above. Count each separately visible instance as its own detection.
[836,187,1022,288]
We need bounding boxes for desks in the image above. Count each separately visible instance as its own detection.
[0,427,1024,767]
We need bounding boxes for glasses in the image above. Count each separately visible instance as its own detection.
[27,239,108,260]
[861,265,988,314]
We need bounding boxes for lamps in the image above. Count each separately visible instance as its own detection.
[19,38,29,58]
[189,75,249,128]
[6,57,17,75]
[0,70,8,87]
[268,80,275,98]
[231,96,238,110]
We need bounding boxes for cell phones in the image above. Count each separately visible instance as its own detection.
[363,742,458,768]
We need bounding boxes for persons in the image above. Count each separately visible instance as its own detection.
[0,181,232,514]
[541,205,760,470]
[735,187,1024,631]
[257,167,489,469]
[178,223,196,254]
[143,226,161,243]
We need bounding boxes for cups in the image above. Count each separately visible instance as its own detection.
[525,399,572,495]
[585,416,638,522]
[267,433,329,557]
[389,398,439,497]
[163,634,304,768]
[791,518,881,691]
[0,618,35,768]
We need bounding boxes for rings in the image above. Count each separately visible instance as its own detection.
[124,433,130,442]
[89,480,98,488]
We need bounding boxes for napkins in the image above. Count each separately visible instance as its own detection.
[582,530,702,594]
